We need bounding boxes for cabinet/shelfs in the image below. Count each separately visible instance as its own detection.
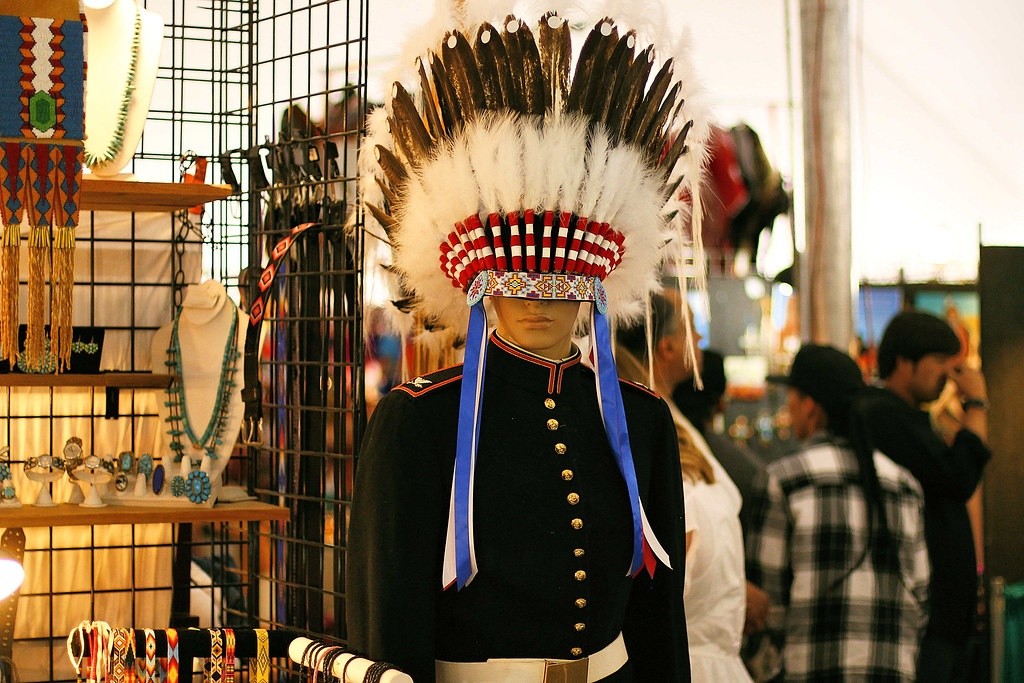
[0,179,291,527]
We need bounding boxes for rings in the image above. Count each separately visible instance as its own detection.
[114,451,211,504]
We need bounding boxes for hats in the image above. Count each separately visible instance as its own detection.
[765,345,863,407]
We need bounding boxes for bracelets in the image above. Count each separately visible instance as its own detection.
[962,398,988,413]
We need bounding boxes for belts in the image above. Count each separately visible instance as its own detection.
[434,631,629,683]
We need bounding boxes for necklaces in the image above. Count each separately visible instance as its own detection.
[298,639,402,683]
[67,619,269,683]
[84,6,142,173]
[164,296,240,467]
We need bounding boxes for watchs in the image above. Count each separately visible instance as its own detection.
[23,454,65,482]
[63,436,84,481]
[71,455,113,484]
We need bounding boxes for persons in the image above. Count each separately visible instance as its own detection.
[617,346,754,683]
[672,350,759,488]
[739,341,931,683]
[868,309,993,683]
[618,286,703,393]
[345,13,694,683]
